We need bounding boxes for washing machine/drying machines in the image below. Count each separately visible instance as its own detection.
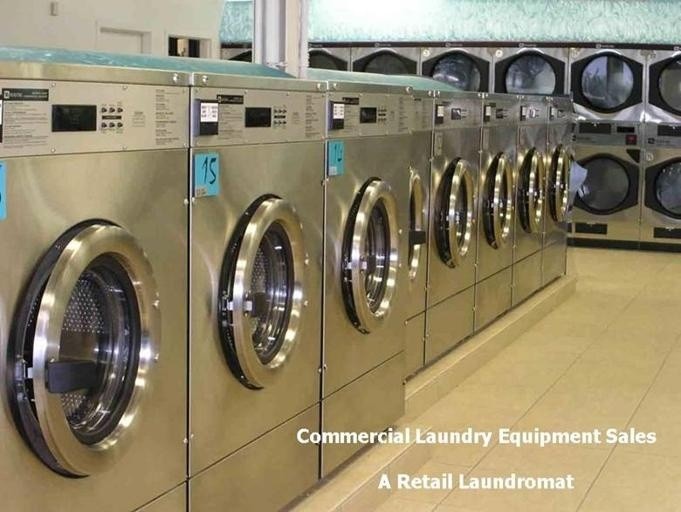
[0,44,680,512]
